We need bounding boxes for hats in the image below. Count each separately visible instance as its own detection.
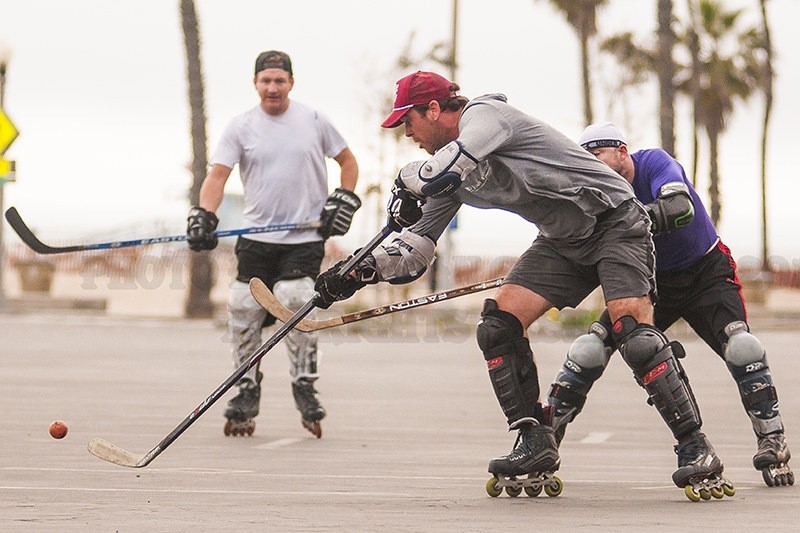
[578,122,627,150]
[381,70,457,128]
[255,50,292,73]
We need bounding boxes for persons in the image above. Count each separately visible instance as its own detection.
[186,51,361,438]
[310,69,735,502]
[541,122,794,487]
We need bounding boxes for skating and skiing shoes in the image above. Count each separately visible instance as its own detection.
[671,433,736,502]
[486,406,563,498]
[291,373,326,439]
[753,431,795,486]
[223,371,263,436]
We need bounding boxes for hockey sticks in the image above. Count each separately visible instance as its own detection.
[86,225,394,469]
[250,274,506,333]
[4,206,323,255]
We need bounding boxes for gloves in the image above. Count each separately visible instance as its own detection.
[313,247,379,310]
[317,188,362,238]
[187,207,220,251]
[386,169,426,233]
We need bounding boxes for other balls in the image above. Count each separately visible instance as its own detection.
[48,420,69,439]
